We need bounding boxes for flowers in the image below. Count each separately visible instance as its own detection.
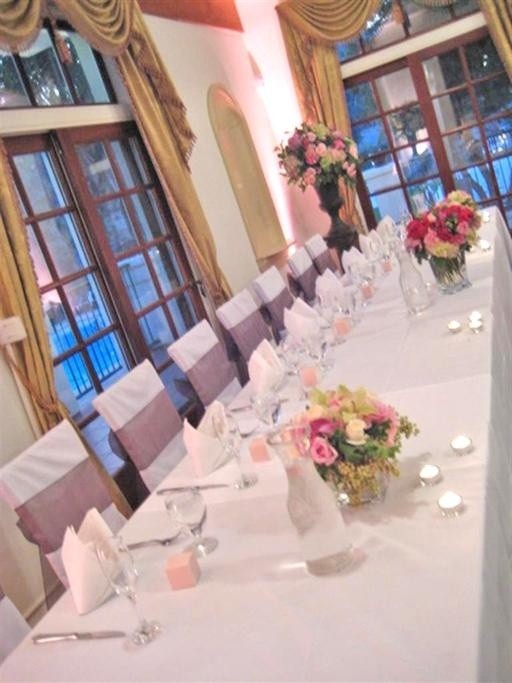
[400,201,483,259]
[271,116,360,186]
[448,188,476,209]
[287,385,420,501]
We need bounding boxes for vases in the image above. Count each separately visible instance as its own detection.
[351,468,391,505]
[319,196,357,249]
[431,260,466,293]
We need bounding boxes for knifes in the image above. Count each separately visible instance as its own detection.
[32,630,126,644]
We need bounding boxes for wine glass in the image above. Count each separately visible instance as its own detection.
[93,208,412,491]
[96,535,165,646]
[158,481,223,562]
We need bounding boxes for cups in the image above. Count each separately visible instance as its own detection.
[447,311,482,334]
[417,434,473,517]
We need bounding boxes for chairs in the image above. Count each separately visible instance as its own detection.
[287,247,318,301]
[215,287,275,362]
[1,587,31,666]
[166,318,243,416]
[303,234,338,275]
[1,419,134,586]
[92,358,188,492]
[251,264,295,333]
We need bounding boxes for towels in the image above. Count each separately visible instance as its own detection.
[284,296,320,336]
[315,266,342,299]
[377,215,397,236]
[358,229,382,252]
[183,400,239,477]
[341,246,368,274]
[61,506,125,615]
[247,338,285,388]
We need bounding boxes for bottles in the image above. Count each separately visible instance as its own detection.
[392,242,432,313]
